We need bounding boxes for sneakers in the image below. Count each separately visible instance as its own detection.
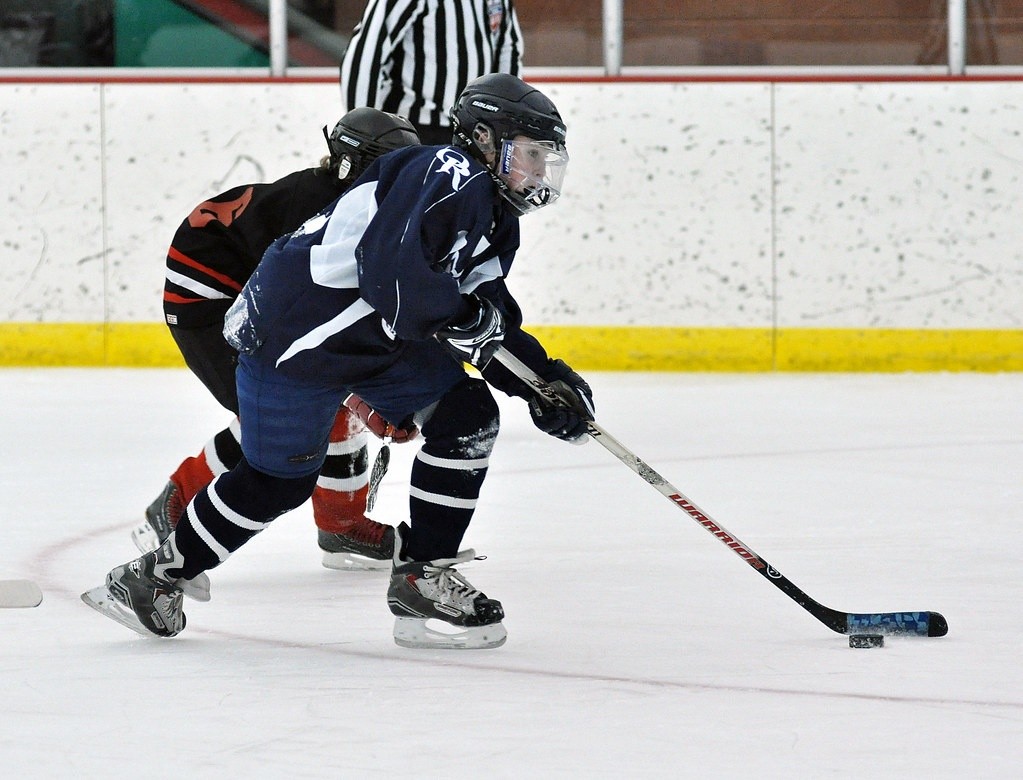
[317,515,394,570]
[386,521,508,649]
[80,531,186,639]
[131,480,211,602]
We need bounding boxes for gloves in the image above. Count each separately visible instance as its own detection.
[432,292,507,372]
[523,358,595,445]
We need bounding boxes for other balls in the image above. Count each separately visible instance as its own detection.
[848,633,884,649]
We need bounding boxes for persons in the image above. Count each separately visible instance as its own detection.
[82,73,596,650]
[339,0,525,145]
[130,108,420,602]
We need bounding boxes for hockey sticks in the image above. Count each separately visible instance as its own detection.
[487,340,954,643]
[1,579,44,609]
[363,421,398,514]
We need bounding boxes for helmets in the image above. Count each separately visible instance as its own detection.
[330,106,422,184]
[448,72,569,217]
